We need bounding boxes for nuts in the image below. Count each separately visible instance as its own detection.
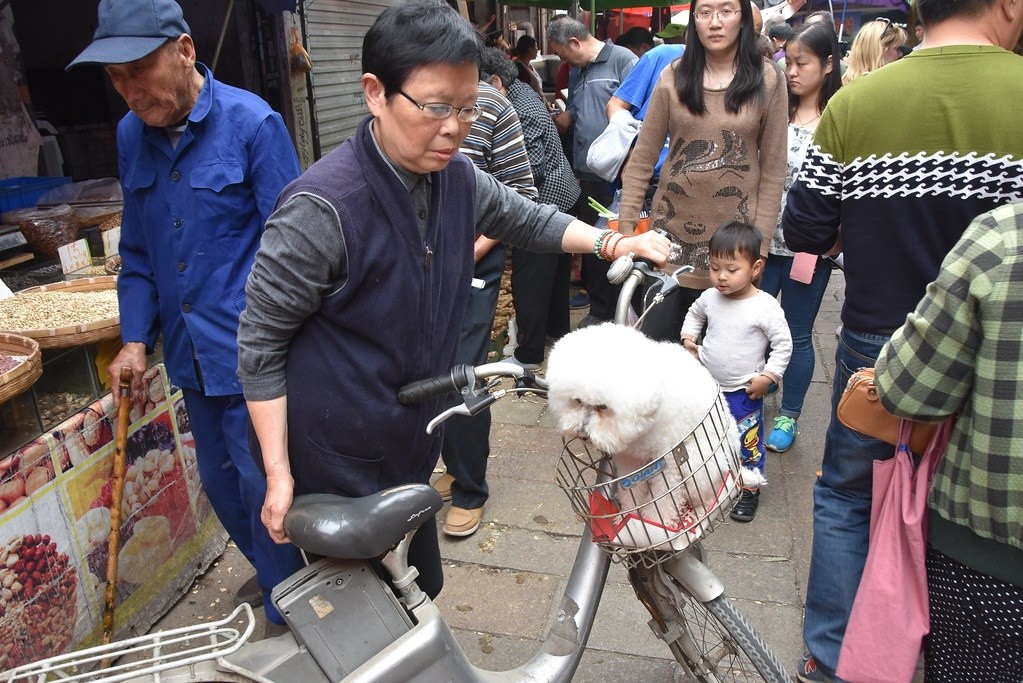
[0,367,208,674]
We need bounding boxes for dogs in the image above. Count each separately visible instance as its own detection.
[546,320,767,544]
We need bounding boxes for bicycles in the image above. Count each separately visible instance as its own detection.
[0,225,794,683]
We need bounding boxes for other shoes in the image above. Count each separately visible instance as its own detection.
[568,279,591,309]
[577,314,614,331]
[264,617,290,639]
[233,575,264,611]
[498,353,542,372]
[544,332,558,343]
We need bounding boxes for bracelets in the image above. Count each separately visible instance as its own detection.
[594,229,629,262]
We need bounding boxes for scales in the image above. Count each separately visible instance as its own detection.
[0,225,29,261]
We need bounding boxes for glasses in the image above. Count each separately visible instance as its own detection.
[875,17,894,40]
[396,88,482,125]
[693,9,742,20]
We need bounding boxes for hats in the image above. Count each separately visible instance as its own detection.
[655,23,686,39]
[64,0,192,75]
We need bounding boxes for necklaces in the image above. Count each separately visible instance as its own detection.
[706,65,737,88]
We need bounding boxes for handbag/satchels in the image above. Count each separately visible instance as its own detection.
[832,412,954,683]
[291,26,313,74]
[836,366,939,459]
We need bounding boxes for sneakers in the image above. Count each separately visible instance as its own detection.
[766,416,797,453]
[797,652,844,682]
[730,487,760,522]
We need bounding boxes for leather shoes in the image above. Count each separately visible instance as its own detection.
[443,498,484,537]
[433,472,456,501]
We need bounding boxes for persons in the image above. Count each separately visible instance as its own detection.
[759,22,844,453]
[235,0,671,625]
[480,49,583,374]
[680,222,794,522]
[874,200,1023,683]
[618,0,791,342]
[510,28,924,202]
[64,0,307,638]
[783,0,1023,683]
[432,78,539,538]
[545,16,638,328]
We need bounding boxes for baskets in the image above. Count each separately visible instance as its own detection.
[552,376,745,570]
[0,254,122,406]
